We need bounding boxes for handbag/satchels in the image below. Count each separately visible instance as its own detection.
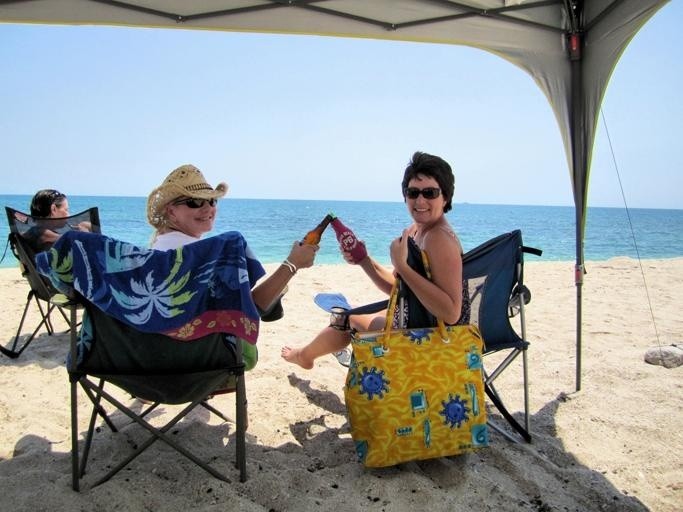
[344,324,488,468]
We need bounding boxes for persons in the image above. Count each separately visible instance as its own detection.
[278,150,471,372]
[19,188,92,260]
[130,163,320,404]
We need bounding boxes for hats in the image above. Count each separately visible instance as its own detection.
[146,164,229,225]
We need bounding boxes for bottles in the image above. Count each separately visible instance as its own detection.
[299,213,335,247]
[329,212,367,266]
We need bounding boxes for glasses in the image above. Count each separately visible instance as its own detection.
[404,187,441,199]
[50,192,60,205]
[171,198,217,209]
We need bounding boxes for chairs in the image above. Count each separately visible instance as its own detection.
[311,228,543,445]
[68,229,249,493]
[0,207,101,360]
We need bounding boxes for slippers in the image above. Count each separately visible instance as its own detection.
[332,348,351,368]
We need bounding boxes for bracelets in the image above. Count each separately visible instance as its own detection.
[280,257,297,274]
